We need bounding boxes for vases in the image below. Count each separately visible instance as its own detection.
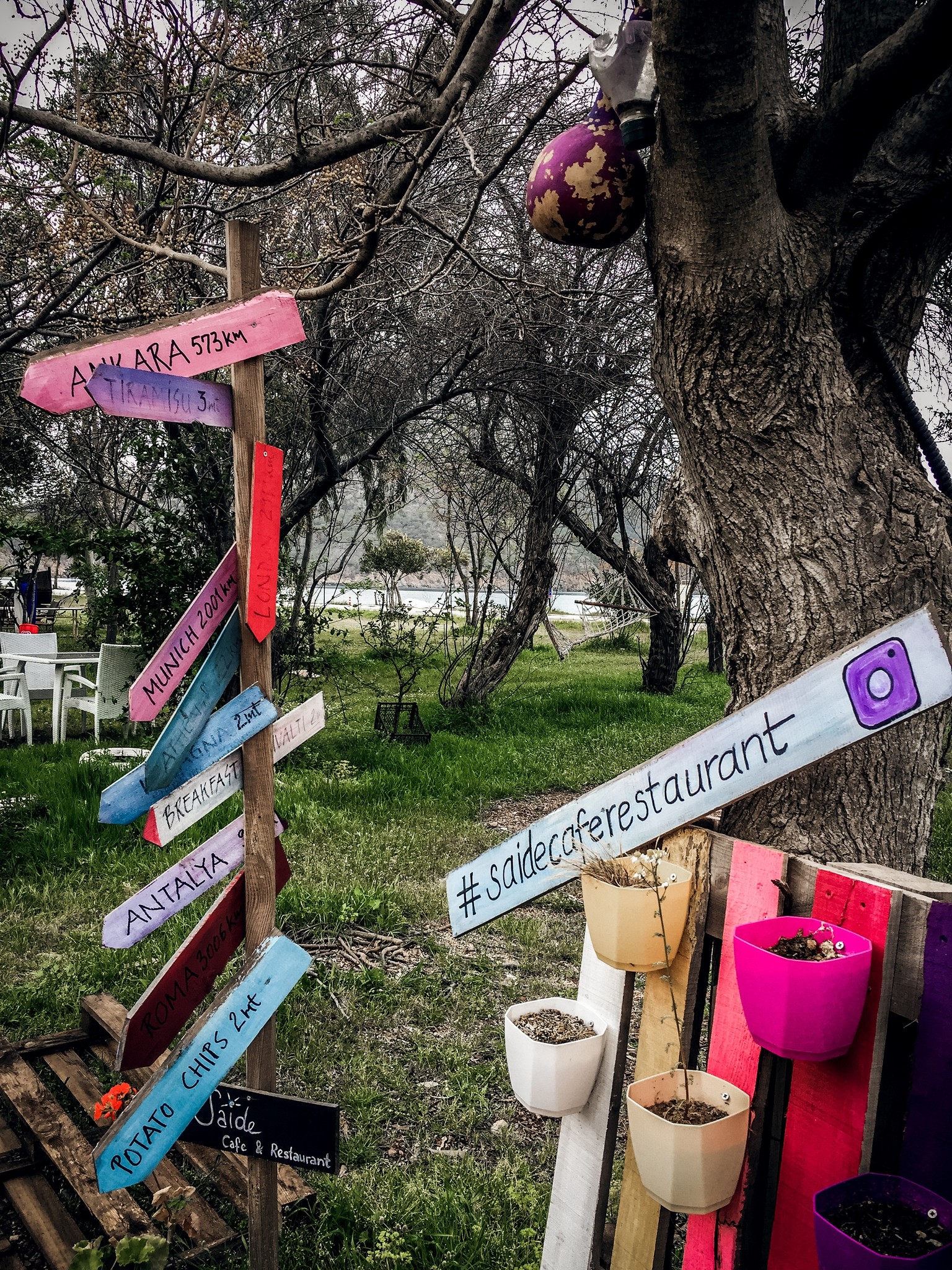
[581,856,693,972]
[628,1070,751,1215]
[504,997,608,1117]
[812,1173,952,1270]
[734,916,873,1059]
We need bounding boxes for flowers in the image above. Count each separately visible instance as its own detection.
[631,848,693,1117]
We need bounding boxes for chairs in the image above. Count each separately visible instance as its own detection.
[35,598,62,631]
[0,632,156,744]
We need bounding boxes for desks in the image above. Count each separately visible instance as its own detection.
[35,607,85,637]
[0,653,103,746]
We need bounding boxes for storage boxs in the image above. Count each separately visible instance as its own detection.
[375,701,432,748]
